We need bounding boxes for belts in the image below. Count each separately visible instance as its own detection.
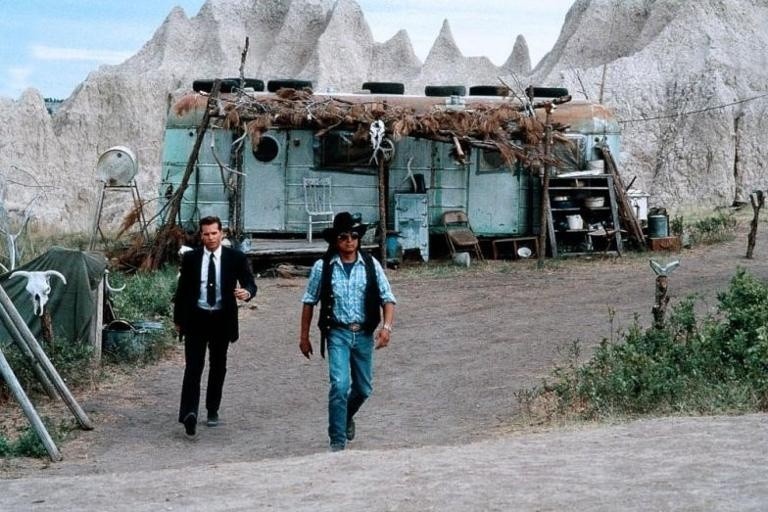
[335,320,365,332]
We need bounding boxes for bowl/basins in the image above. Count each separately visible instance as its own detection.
[552,200,572,209]
[585,198,605,209]
[517,246,532,259]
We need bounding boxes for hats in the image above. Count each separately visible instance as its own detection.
[322,212,368,245]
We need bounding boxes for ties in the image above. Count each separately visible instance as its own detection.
[207,251,216,307]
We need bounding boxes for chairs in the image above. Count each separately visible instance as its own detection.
[442,210,484,262]
[301,176,335,244]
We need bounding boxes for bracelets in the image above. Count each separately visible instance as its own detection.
[382,324,393,334]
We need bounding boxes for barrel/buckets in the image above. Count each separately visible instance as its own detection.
[102,322,147,364]
[648,214,668,238]
[518,248,532,260]
[451,252,470,269]
[96,145,137,188]
[385,232,403,262]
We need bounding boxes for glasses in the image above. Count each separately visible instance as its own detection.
[337,231,359,241]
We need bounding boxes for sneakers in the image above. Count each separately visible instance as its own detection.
[346,419,355,440]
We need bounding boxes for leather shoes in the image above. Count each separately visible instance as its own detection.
[206,414,219,426]
[183,413,197,435]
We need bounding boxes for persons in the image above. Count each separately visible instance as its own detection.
[173,216,257,436]
[300,212,396,451]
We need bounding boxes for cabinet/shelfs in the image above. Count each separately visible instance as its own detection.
[393,193,430,263]
[540,174,625,259]
